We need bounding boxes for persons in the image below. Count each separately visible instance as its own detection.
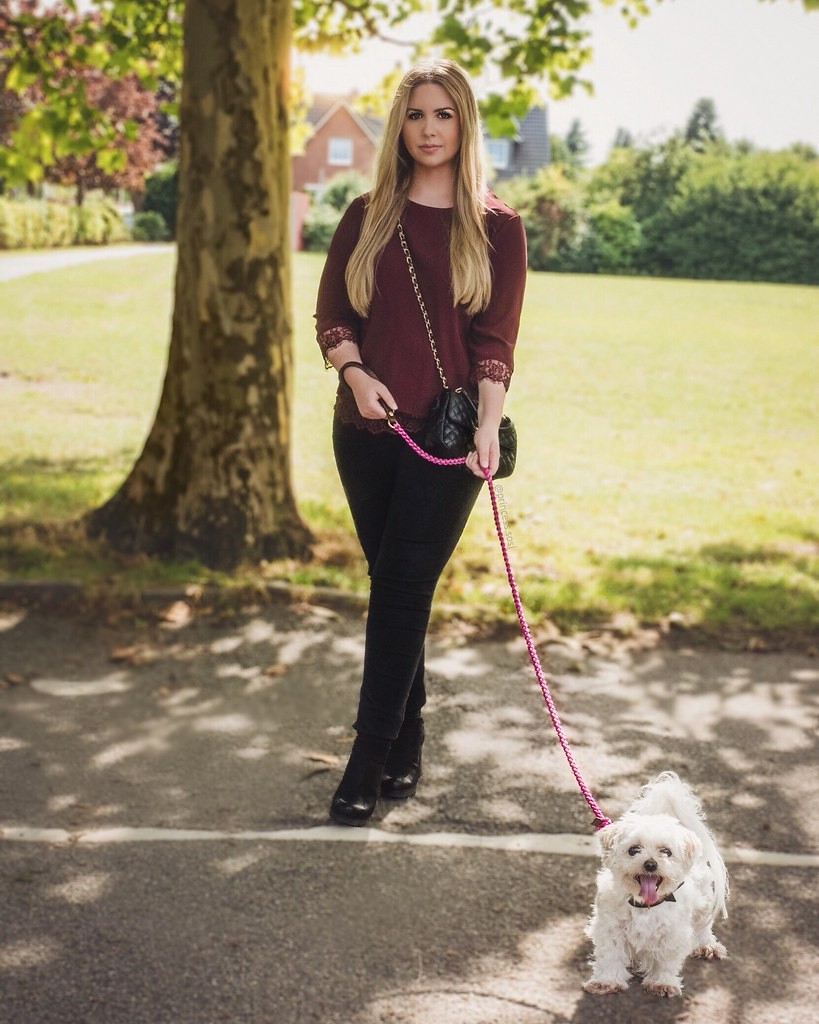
[316,61,528,825]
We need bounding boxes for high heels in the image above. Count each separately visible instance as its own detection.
[329,737,391,828]
[380,715,425,798]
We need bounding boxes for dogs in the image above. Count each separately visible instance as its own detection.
[579,770,736,998]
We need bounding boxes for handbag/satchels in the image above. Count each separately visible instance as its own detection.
[420,387,517,480]
